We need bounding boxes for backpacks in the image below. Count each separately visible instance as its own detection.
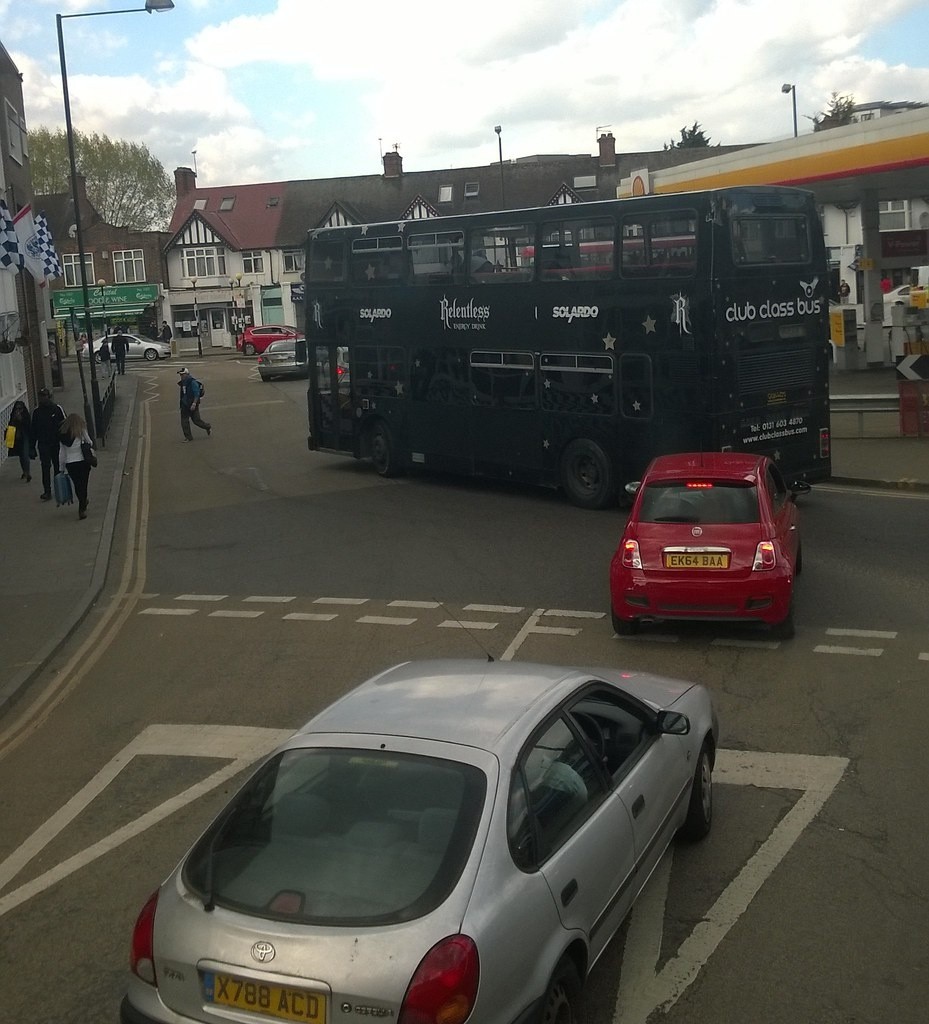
[188,378,205,397]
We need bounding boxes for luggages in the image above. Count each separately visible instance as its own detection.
[54,473,74,507]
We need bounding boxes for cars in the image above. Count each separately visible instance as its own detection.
[119,658,717,1024]
[257,338,308,381]
[237,325,305,356]
[883,284,911,305]
[610,451,812,635]
[82,333,172,364]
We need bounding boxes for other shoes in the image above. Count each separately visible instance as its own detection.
[20,474,25,480]
[86,499,89,506]
[207,424,212,436]
[108,376,112,379]
[118,372,125,375]
[26,476,32,482]
[79,511,87,519]
[40,491,51,499]
[102,377,105,380]
[181,436,193,443]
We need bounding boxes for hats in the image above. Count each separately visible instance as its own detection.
[38,388,49,395]
[176,367,189,375]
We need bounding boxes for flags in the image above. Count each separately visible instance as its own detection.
[0,198,25,276]
[13,204,47,289]
[33,212,63,281]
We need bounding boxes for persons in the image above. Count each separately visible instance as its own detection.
[4,401,38,483]
[59,413,96,520]
[111,328,129,375]
[450,249,494,274]
[839,279,850,305]
[176,367,212,443]
[99,339,111,379]
[162,321,172,344]
[29,388,67,499]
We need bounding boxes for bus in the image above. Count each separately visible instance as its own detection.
[304,185,833,508]
[521,235,696,272]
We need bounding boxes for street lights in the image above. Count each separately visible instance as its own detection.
[782,84,798,137]
[190,276,202,356]
[236,272,246,356]
[228,278,238,344]
[56,0,176,438]
[495,126,505,211]
[97,279,110,348]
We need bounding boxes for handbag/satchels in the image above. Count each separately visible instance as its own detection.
[4,426,16,448]
[81,433,97,468]
